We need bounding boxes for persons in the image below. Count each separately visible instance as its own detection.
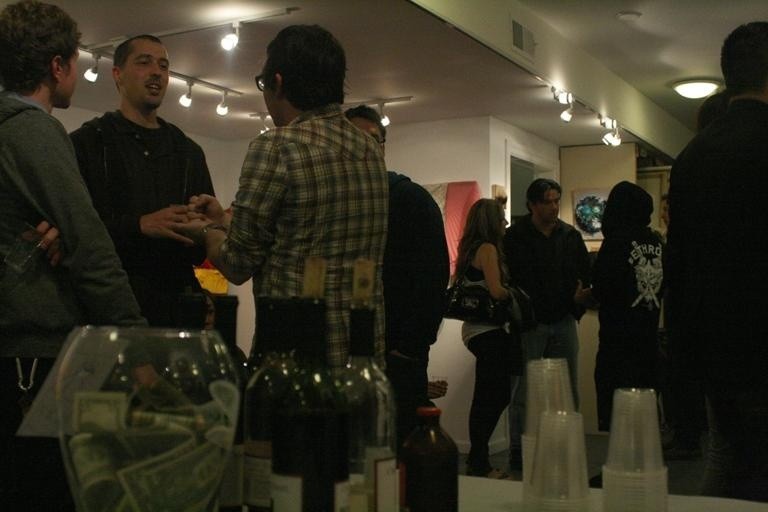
[659,20,767,501]
[68,35,221,410]
[175,24,390,510]
[0,0,173,511]
[344,105,449,455]
[456,179,668,483]
[187,288,248,444]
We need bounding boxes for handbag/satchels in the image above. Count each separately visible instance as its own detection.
[442,284,507,326]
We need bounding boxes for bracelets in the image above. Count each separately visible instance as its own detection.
[198,223,228,240]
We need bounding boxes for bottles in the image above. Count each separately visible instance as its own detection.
[397,401,462,508]
[242,286,395,510]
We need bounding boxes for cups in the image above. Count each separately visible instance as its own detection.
[597,386,672,510]
[56,323,243,512]
[519,358,570,479]
[520,411,590,511]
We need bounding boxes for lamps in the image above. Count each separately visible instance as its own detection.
[85,6,290,51]
[247,97,411,136]
[672,80,722,101]
[76,45,244,115]
[543,79,625,149]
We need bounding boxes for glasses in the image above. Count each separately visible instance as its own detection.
[253,72,273,91]
